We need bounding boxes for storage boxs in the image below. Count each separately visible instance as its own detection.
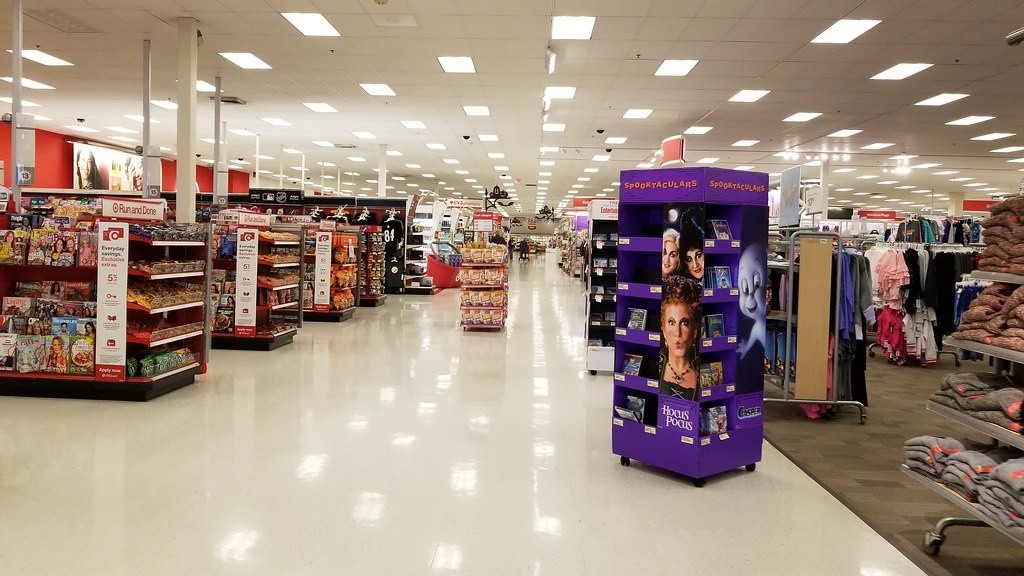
[407,235,423,245]
[420,277,433,286]
[410,251,423,260]
[412,278,420,282]
[411,282,420,287]
[153,346,196,368]
[411,266,419,273]
[0,206,98,377]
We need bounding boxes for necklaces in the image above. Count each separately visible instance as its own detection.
[666,362,692,380]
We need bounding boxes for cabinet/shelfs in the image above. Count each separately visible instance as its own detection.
[0,196,213,403]
[338,225,388,308]
[271,220,362,323]
[459,254,508,331]
[762,231,868,425]
[571,216,588,278]
[211,208,305,352]
[583,199,619,376]
[611,166,769,490]
[900,270,1024,557]
[162,188,438,296]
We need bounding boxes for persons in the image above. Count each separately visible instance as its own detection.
[228,286,235,294]
[226,296,234,308]
[508,237,516,259]
[31,320,43,334]
[76,145,104,190]
[63,307,77,317]
[211,284,219,294]
[65,238,77,255]
[85,307,92,318]
[2,231,16,252]
[38,235,47,253]
[490,231,506,245]
[82,322,96,340]
[50,282,60,295]
[125,155,142,191]
[659,206,705,401]
[53,238,66,254]
[92,306,97,318]
[47,336,67,368]
[519,237,528,261]
[58,322,70,335]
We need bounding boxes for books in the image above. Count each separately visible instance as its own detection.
[0,281,97,375]
[614,308,648,423]
[0,229,98,267]
[209,269,236,335]
[697,220,733,435]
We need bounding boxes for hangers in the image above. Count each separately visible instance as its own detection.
[955,273,994,289]
[310,206,323,217]
[870,242,987,256]
[384,207,400,222]
[903,213,948,223]
[327,206,347,221]
[357,207,371,221]
